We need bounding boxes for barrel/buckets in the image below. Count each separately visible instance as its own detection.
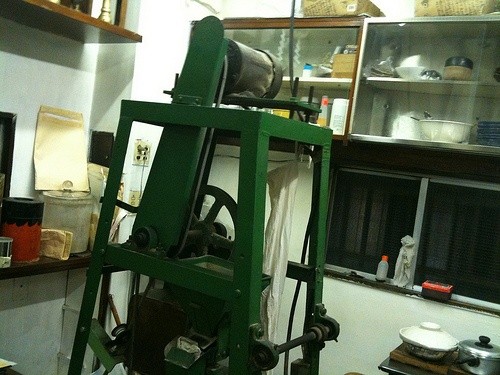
[42,191,92,253]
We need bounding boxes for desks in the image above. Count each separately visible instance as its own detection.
[379,343,434,375]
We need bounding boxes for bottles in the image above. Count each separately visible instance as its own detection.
[317,99,328,126]
[303,63,313,78]
[376,255,389,280]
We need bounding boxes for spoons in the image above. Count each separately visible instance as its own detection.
[423,110,432,119]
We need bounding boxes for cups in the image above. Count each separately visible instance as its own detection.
[0,237,13,268]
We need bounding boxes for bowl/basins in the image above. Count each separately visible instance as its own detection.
[395,66,427,80]
[419,70,441,80]
[400,55,431,66]
[444,56,474,80]
[419,120,473,143]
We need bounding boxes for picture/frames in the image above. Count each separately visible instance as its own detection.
[0,111,17,211]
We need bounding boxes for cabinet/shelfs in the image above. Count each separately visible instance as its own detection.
[218,18,500,155]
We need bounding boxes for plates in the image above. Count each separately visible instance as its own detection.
[477,121,500,146]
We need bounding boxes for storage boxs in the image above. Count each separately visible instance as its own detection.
[302,0,380,19]
[332,54,356,79]
[414,0,500,17]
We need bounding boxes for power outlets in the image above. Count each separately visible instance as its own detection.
[134,139,151,167]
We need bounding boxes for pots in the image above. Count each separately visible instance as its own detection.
[458,335,500,375]
[398,321,459,359]
